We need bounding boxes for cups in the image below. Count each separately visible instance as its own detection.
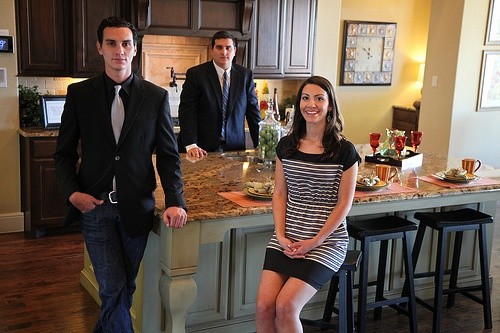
[462,158,481,175]
[376,164,398,186]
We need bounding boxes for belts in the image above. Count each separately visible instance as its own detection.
[99,192,119,204]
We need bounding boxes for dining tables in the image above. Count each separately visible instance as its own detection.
[76,141,500,333]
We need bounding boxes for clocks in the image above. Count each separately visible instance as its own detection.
[339,19,399,87]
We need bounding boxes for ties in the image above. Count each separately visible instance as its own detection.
[221,71,229,140]
[111,85,125,191]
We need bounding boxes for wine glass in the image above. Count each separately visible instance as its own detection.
[385,129,407,162]
[410,130,423,153]
[369,133,381,157]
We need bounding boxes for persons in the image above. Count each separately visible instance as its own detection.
[256,76,361,333]
[54,17,187,333]
[177,31,261,158]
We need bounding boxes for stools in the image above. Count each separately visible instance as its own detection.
[300,248,363,333]
[322,215,419,333]
[399,208,495,333]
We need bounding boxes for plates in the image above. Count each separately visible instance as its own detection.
[436,170,479,182]
[356,180,387,190]
[243,187,273,199]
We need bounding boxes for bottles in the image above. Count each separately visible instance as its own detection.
[257,102,282,163]
[272,88,281,121]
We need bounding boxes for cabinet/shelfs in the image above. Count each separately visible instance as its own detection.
[15,0,315,91]
[19,135,67,240]
